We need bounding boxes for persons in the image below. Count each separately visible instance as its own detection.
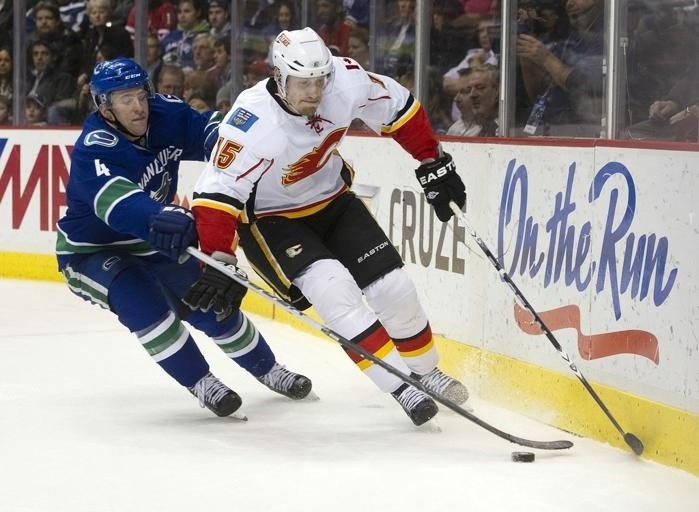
[625,2,698,142]
[1,2,135,128]
[517,0,606,138]
[126,0,504,136]
[191,25,470,426]
[55,57,311,417]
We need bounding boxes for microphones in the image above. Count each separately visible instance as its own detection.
[572,3,598,20]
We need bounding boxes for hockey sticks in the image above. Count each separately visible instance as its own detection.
[449,201,644,453]
[186,244,581,449]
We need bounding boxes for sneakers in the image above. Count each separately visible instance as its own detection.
[392,367,470,426]
[258,361,311,398]
[188,373,241,416]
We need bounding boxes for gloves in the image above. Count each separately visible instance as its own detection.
[183,260,248,321]
[148,205,199,264]
[415,154,465,223]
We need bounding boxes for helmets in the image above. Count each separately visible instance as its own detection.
[91,57,156,110]
[271,27,336,95]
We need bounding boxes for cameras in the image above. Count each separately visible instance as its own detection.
[486,17,547,55]
